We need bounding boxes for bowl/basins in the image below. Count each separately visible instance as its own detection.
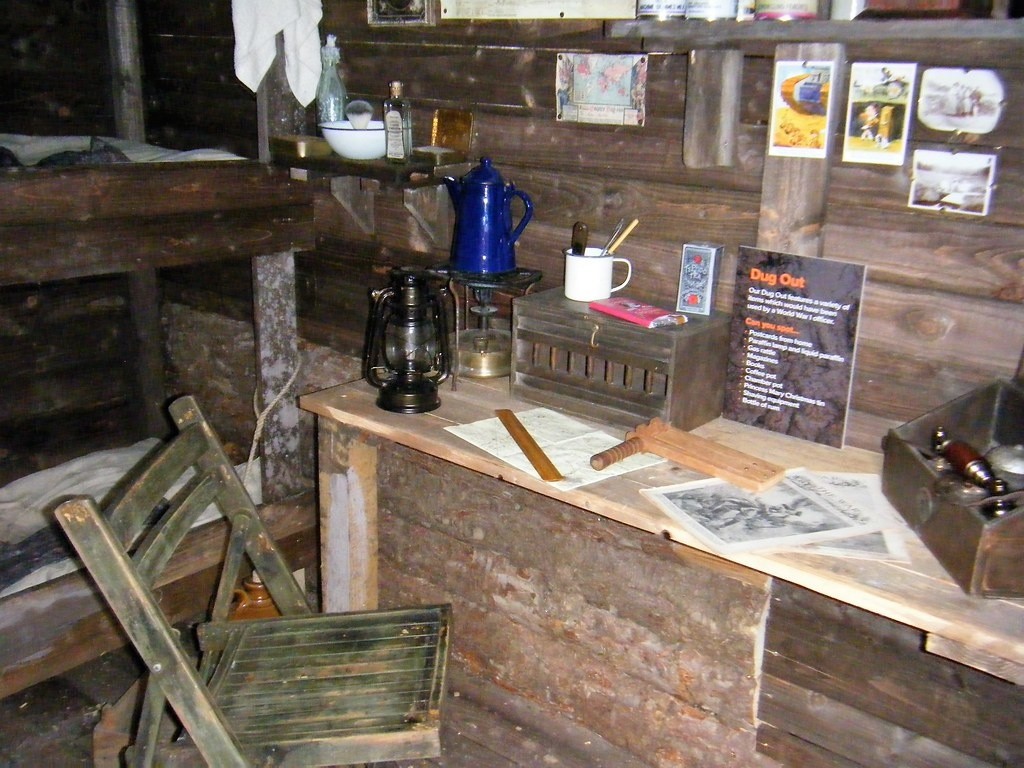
[318,120,386,159]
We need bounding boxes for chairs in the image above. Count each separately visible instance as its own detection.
[54,394,452,768]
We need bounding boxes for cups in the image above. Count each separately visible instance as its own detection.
[563,247,631,302]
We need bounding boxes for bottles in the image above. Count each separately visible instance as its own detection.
[316,46,347,122]
[384,80,412,163]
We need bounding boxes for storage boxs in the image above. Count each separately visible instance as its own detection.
[879,376,1023,601]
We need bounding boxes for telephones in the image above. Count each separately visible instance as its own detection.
[929,425,1018,520]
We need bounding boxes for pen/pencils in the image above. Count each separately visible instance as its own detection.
[599,217,624,257]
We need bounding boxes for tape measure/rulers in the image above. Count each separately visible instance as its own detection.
[494,409,563,482]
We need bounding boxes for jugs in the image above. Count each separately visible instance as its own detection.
[440,157,533,276]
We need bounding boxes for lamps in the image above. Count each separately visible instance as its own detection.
[360,271,451,415]
